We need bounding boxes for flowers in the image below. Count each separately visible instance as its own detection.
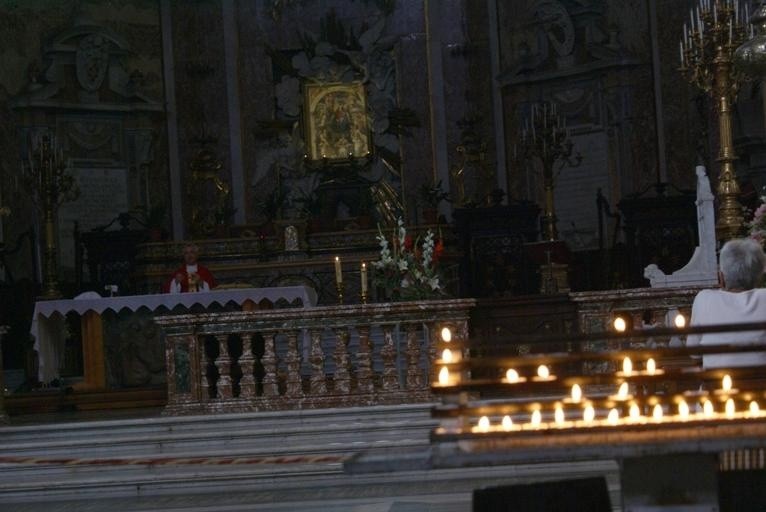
[369,217,453,304]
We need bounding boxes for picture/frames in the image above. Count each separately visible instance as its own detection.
[301,79,375,164]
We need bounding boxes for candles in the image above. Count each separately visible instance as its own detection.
[14,126,71,192]
[679,0,754,68]
[511,98,584,158]
[360,263,368,291]
[334,256,343,282]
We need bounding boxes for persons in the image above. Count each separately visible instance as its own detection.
[686,240,766,370]
[161,244,217,293]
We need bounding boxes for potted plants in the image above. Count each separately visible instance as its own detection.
[417,178,456,223]
[257,185,327,254]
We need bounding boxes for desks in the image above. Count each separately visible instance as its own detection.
[31,285,317,389]
